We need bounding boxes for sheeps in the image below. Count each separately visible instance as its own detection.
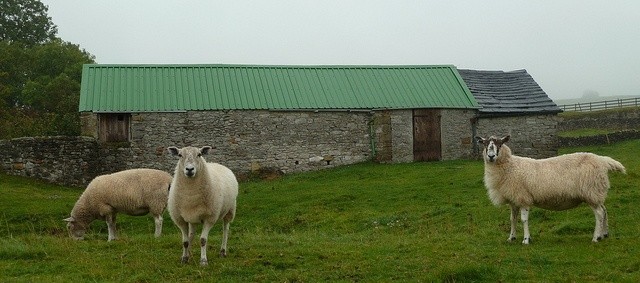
[168,146,239,265]
[474,134,627,242]
[61,169,172,242]
